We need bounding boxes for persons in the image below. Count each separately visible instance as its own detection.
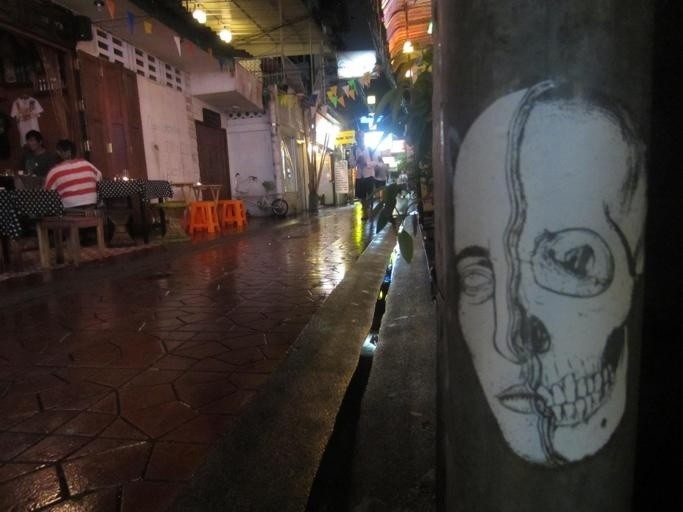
[23,129,61,174]
[347,129,407,220]
[41,140,116,245]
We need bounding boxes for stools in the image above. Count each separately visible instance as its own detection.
[0,173,247,273]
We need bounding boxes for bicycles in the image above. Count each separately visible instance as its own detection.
[233,172,289,218]
